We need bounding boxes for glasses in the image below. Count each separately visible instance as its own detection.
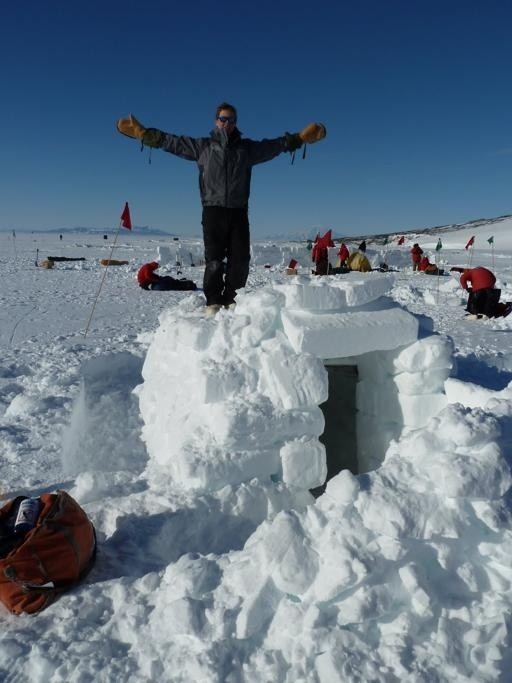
[218,115,236,124]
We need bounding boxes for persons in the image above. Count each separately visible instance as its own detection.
[138,262,163,291]
[337,242,350,269]
[115,102,328,317]
[459,265,502,318]
[410,244,423,272]
[347,240,371,271]
[312,246,332,274]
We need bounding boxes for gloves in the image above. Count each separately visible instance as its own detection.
[300,121,326,143]
[116,111,145,140]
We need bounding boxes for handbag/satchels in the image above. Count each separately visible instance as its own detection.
[0,492,97,614]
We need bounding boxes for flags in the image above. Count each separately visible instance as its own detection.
[436,238,442,252]
[383,238,388,247]
[487,237,494,246]
[398,236,405,246]
[305,240,314,251]
[313,229,331,260]
[465,237,475,250]
[122,202,133,232]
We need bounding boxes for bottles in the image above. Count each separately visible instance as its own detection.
[12,496,43,546]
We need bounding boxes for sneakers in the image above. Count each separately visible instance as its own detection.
[139,284,150,290]
[206,303,236,316]
